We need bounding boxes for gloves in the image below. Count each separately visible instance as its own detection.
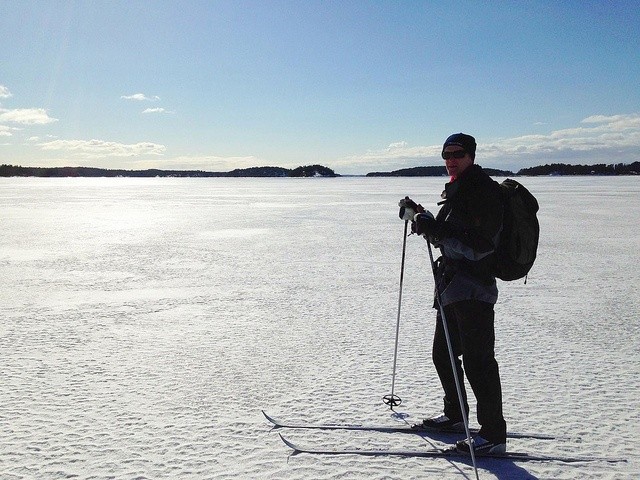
[398,199,426,237]
[411,213,442,247]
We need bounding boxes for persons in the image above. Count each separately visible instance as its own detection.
[398,132,506,455]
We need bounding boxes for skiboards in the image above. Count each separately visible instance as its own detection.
[261,410,629,463]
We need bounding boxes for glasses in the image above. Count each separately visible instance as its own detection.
[442,150,469,159]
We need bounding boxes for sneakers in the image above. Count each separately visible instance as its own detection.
[456,434,506,455]
[423,413,468,429]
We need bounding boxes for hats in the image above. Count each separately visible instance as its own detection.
[443,133,476,159]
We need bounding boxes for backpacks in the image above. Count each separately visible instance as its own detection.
[496,178,539,284]
